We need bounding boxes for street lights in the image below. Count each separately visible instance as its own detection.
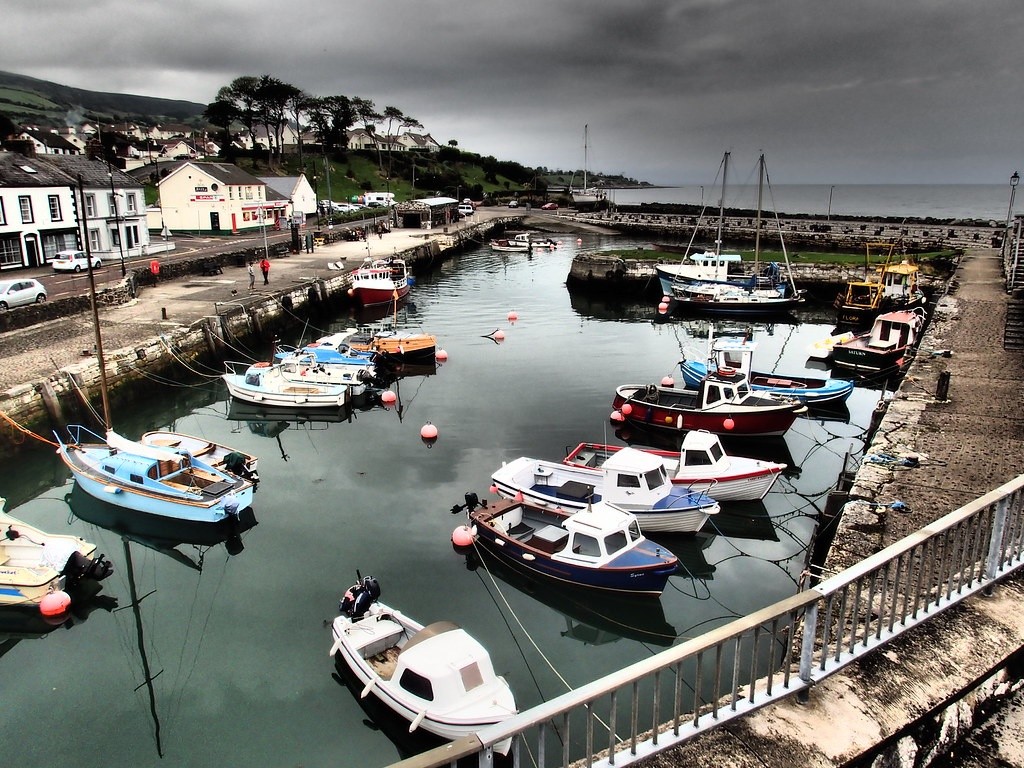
[1006,170,1021,229]
[456,185,462,200]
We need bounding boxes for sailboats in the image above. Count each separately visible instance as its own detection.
[569,122,609,209]
[53,172,255,527]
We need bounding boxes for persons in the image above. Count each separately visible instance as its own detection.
[342,227,367,242]
[260,257,270,285]
[248,262,256,289]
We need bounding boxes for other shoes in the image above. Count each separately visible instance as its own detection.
[248,287,251,290]
[252,287,256,289]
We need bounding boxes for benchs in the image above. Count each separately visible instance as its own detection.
[202,260,223,275]
[356,620,405,658]
[571,450,597,468]
[160,467,224,494]
[555,541,581,554]
[275,246,291,259]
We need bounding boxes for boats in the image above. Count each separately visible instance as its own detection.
[490,446,726,539]
[221,317,437,413]
[560,427,787,510]
[329,568,524,761]
[348,241,416,311]
[142,430,260,484]
[449,491,680,602]
[488,231,559,253]
[0,497,114,619]
[610,147,938,445]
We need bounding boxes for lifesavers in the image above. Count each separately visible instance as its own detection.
[718,365,736,377]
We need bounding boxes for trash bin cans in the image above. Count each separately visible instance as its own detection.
[426,221,431,230]
[314,232,322,242]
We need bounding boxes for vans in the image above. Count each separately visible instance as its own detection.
[362,191,399,209]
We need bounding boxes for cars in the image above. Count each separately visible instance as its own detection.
[0,279,48,316]
[318,198,386,216]
[508,200,519,207]
[542,202,558,210]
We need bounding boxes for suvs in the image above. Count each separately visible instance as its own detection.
[457,198,477,215]
[52,250,103,274]
